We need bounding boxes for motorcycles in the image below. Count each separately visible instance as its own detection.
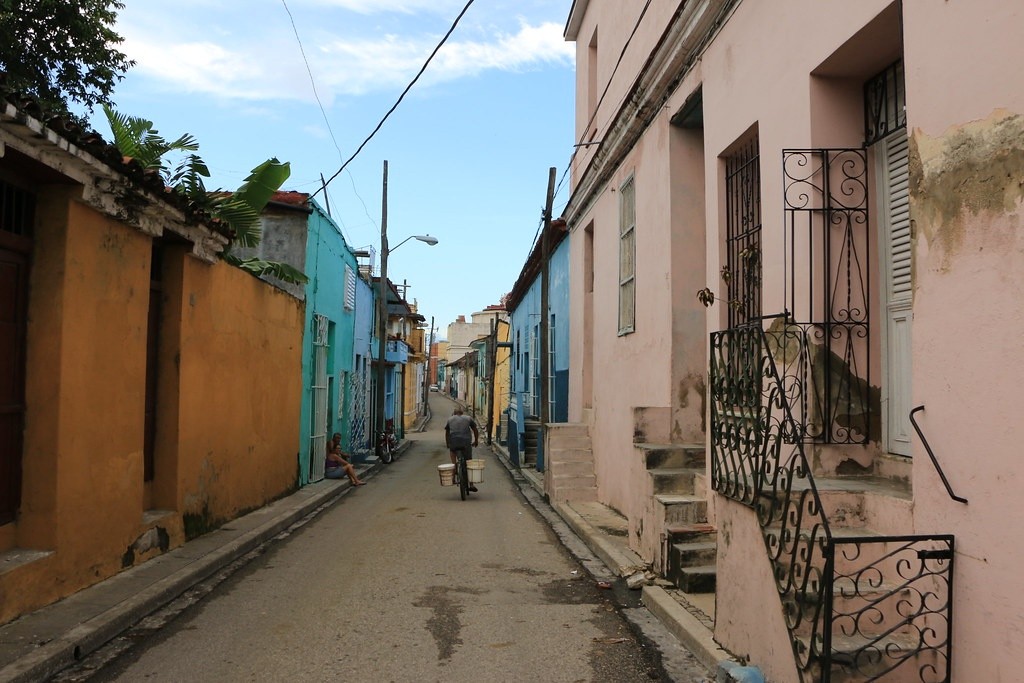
[374,425,398,465]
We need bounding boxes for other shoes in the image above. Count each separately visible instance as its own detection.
[354,481,366,486]
[470,486,478,492]
[351,481,356,486]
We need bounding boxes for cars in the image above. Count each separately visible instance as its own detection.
[430,384,439,392]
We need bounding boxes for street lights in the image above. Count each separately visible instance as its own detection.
[375,236,439,456]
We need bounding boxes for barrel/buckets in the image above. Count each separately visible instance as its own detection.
[437,464,455,486]
[467,459,485,482]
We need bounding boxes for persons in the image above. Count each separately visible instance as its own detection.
[325,433,367,486]
[445,408,479,492]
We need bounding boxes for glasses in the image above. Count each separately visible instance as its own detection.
[336,447,342,451]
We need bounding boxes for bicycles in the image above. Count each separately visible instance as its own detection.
[452,444,476,501]
